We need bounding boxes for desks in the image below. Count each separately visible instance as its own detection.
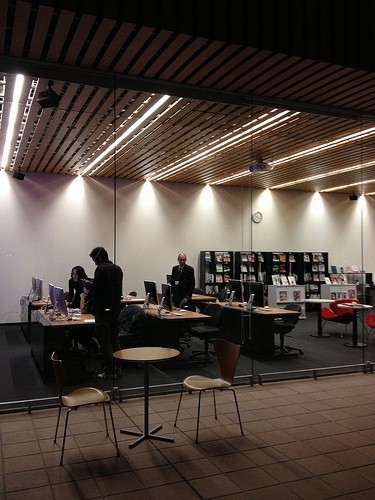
[136,304,212,367]
[191,294,218,301]
[113,347,180,449]
[202,302,301,362]
[30,308,96,383]
[337,303,373,348]
[120,295,144,303]
[20,299,51,343]
[305,299,336,338]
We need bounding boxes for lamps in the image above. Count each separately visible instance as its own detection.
[13,166,25,180]
[349,193,358,200]
[36,80,61,108]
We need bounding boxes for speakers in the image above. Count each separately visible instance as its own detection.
[350,195,357,200]
[36,89,60,109]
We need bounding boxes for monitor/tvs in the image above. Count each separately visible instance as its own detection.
[143,280,173,316]
[227,279,264,311]
[28,276,69,321]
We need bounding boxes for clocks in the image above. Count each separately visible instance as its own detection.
[252,212,263,223]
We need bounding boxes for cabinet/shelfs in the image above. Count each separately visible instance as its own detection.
[200,251,372,319]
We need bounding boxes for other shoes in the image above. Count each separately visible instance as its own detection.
[97,371,117,378]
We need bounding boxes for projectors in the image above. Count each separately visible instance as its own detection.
[253,163,273,172]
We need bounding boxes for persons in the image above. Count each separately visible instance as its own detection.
[169,253,195,342]
[89,247,123,380]
[64,266,98,356]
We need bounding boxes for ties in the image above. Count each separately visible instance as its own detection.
[176,268,182,279]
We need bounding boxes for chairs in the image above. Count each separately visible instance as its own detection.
[53,281,304,466]
[322,299,375,341]
